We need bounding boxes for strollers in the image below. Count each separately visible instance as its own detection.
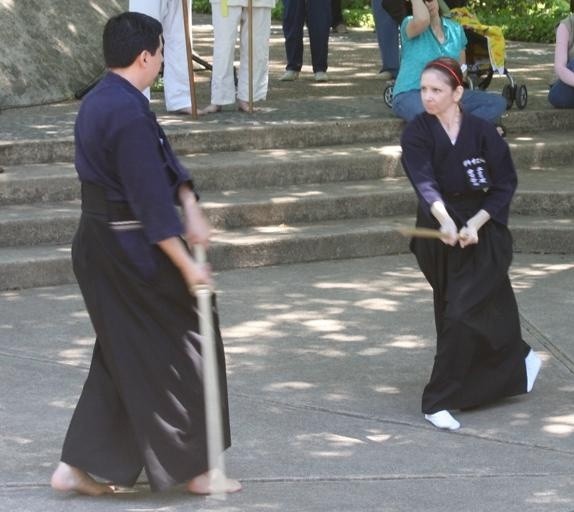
[383,0,527,111]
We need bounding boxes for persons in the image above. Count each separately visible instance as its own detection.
[397,57,545,433]
[199,1,277,116]
[331,0,350,38]
[381,0,507,139]
[276,0,334,84]
[45,10,246,499]
[127,0,202,118]
[371,0,413,80]
[548,0,573,110]
